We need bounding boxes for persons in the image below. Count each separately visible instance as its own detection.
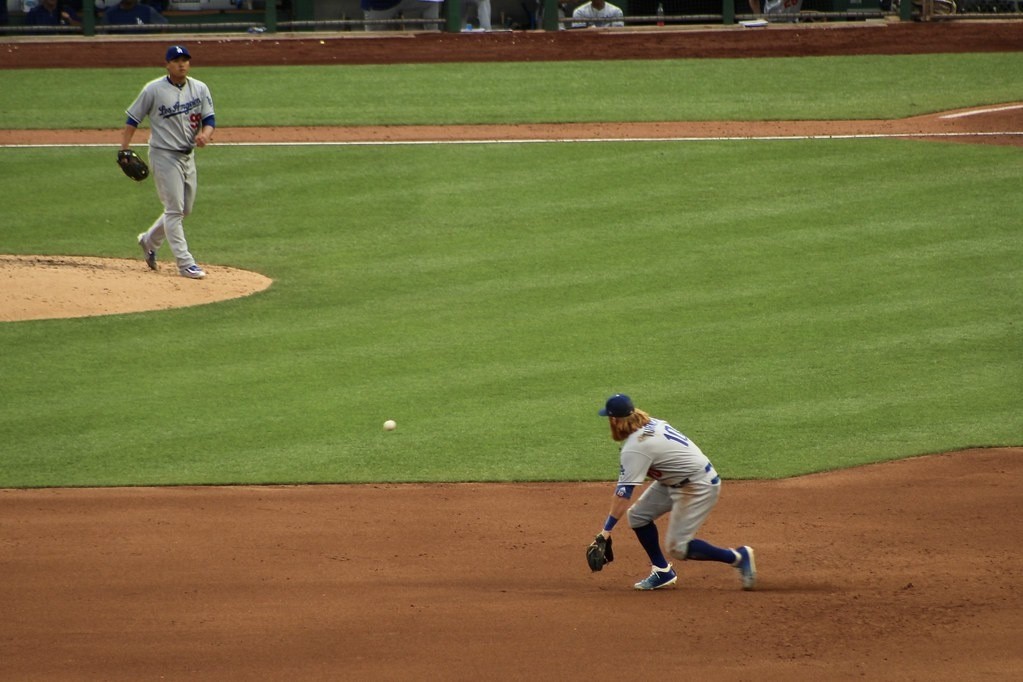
[585,395,757,591]
[360,0,802,33]
[21,0,167,33]
[115,47,216,279]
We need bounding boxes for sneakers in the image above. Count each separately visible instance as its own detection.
[736,546,757,590]
[181,266,206,279]
[634,563,677,590]
[137,234,158,270]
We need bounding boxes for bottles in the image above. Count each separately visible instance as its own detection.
[248,26,266,35]
[657,3,664,26]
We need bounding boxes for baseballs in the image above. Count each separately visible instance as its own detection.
[383,419,397,431]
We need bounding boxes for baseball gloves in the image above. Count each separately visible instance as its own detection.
[584,536,615,572]
[117,150,149,180]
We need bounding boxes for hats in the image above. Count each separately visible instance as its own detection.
[599,394,634,417]
[166,46,191,62]
